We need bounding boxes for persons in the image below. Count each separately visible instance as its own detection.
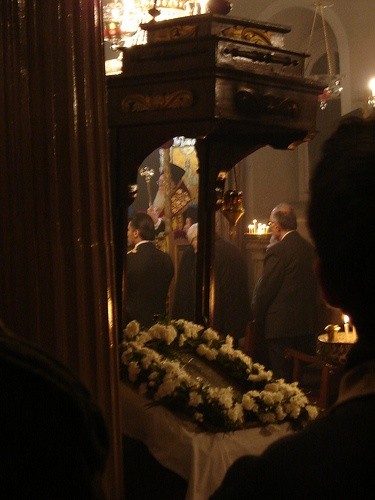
[146,164,193,242]
[208,114,375,500]
[123,202,320,383]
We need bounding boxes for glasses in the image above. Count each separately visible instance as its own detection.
[267,221,279,226]
[189,238,194,248]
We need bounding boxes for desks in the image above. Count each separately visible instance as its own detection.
[316,333,358,398]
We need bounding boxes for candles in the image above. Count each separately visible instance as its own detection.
[247,219,270,235]
[342,315,350,337]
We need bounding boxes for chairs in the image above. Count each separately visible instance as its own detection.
[283,342,319,389]
[239,315,256,356]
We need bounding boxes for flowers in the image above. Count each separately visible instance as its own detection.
[121,318,319,432]
